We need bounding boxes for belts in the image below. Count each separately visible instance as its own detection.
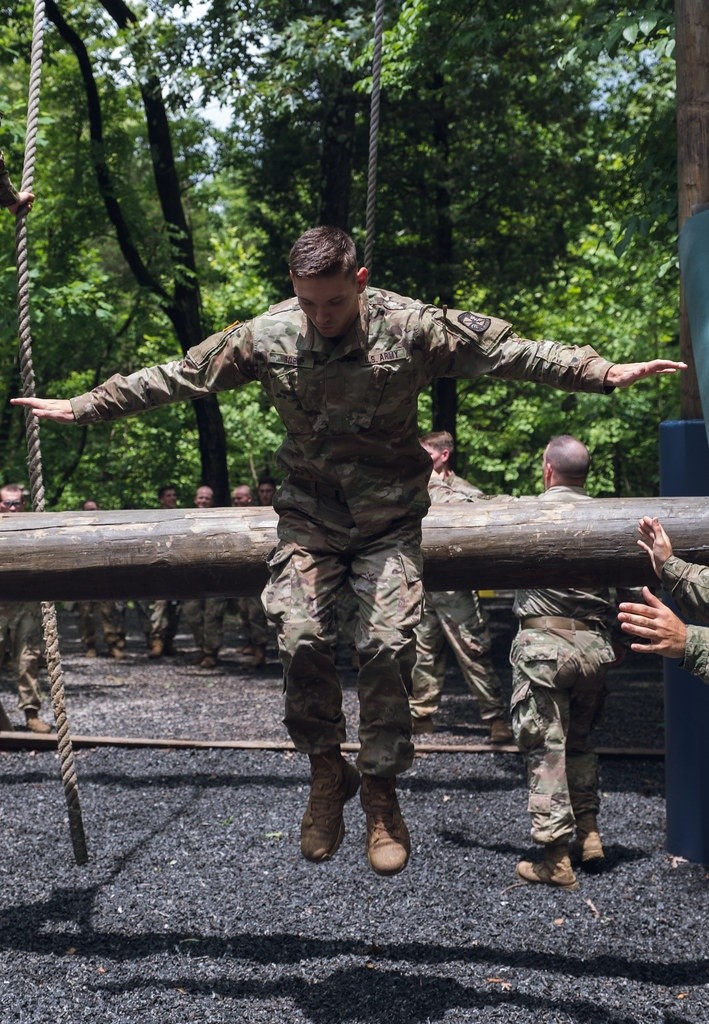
[521,616,594,631]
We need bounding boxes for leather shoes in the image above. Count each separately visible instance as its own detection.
[574,812,604,863]
[360,781,412,876]
[517,837,579,891]
[299,755,361,863]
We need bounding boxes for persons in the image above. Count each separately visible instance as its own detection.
[0,431,618,885]
[10,227,689,876]
[618,515,709,685]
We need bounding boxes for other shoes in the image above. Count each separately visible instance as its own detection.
[24,708,51,734]
[200,656,218,667]
[490,718,516,745]
[107,645,125,658]
[413,714,435,736]
[86,647,96,658]
[236,644,254,654]
[250,643,267,666]
[148,638,177,659]
